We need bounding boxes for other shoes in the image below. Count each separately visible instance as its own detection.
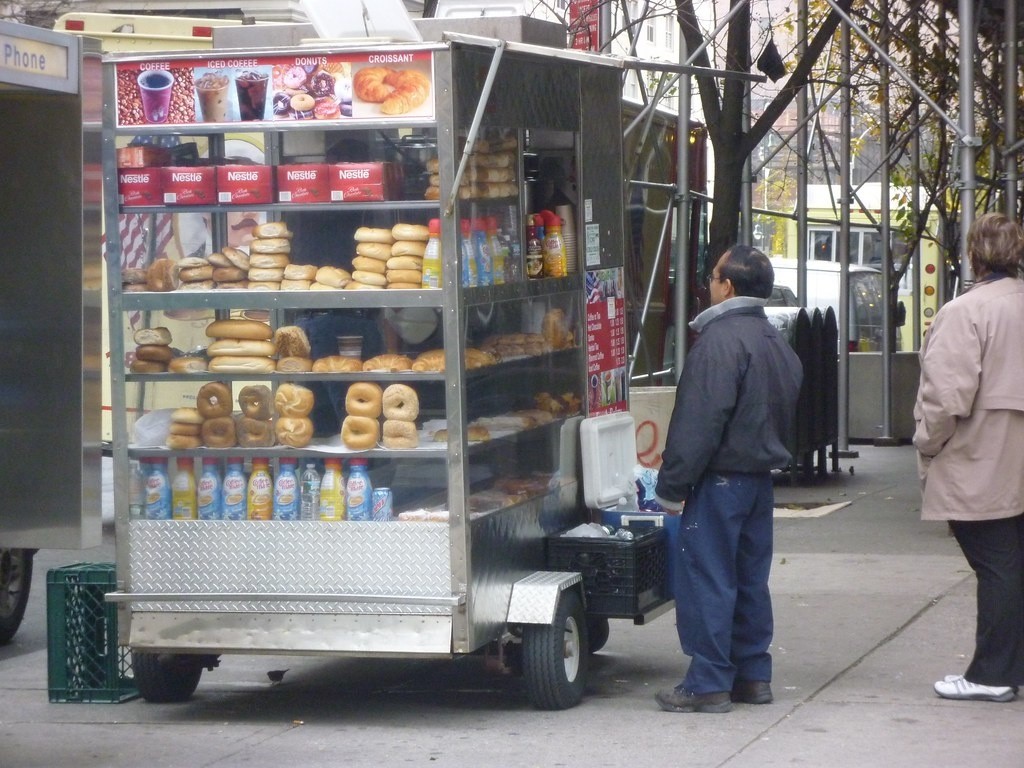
[731,680,774,703]
[655,684,732,713]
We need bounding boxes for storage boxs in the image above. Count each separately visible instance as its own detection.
[46,561,146,706]
[546,409,685,619]
[116,146,407,206]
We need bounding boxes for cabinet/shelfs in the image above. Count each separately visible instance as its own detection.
[98,45,622,654]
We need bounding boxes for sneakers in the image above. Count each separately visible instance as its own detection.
[934,675,1014,702]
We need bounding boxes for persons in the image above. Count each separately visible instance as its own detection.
[913,212,1024,701]
[653,245,803,713]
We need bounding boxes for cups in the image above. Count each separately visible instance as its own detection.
[137,69,175,123]
[554,204,577,272]
[193,77,231,123]
[234,74,270,122]
[335,334,364,360]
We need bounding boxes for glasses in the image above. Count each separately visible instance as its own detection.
[706,274,722,284]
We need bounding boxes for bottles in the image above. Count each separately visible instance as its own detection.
[421,209,569,291]
[129,455,374,522]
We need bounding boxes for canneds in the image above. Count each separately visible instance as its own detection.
[372,487,394,521]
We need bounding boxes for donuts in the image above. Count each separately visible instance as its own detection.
[272,63,351,120]
[197,382,419,450]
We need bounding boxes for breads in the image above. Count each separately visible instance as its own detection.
[352,67,429,115]
[434,390,582,442]
[423,134,520,200]
[397,476,541,522]
[166,406,203,449]
[121,221,432,290]
[128,308,574,373]
